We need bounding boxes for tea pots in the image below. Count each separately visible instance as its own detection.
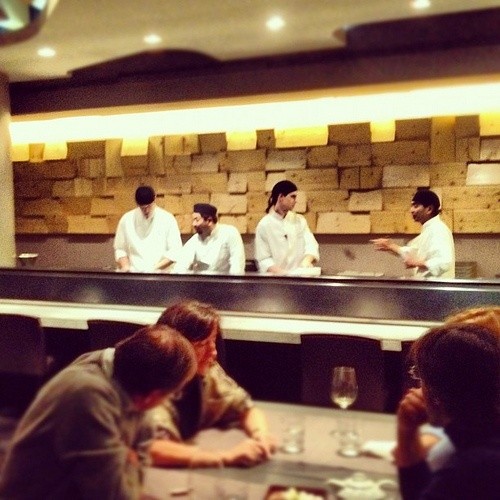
[329,471,398,500]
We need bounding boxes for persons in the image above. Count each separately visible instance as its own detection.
[132,300,270,467]
[0,324,198,500]
[172,203,246,275]
[369,190,455,279]
[393,305,500,500]
[255,180,320,274]
[114,186,183,273]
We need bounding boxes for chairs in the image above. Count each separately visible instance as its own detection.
[82,318,146,357]
[300,332,392,412]
[400,339,419,407]
[0,312,65,390]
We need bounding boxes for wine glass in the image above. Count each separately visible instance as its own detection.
[329,366,359,438]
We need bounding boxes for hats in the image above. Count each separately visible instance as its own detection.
[194,203,217,217]
[271,180,296,205]
[412,192,440,209]
[135,186,155,205]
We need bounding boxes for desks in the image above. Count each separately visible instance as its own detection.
[142,400,399,500]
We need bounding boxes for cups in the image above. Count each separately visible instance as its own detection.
[338,416,364,457]
[277,413,305,453]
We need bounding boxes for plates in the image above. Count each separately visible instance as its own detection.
[263,483,328,500]
